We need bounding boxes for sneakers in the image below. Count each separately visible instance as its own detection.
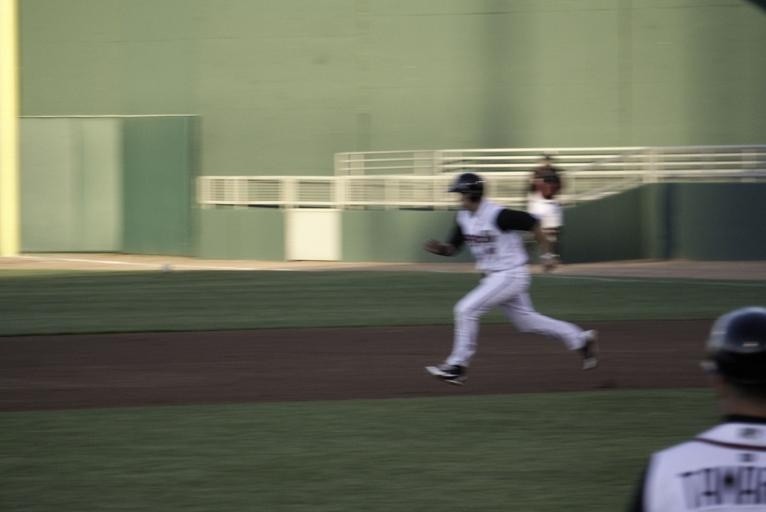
[581,330,601,370]
[426,363,468,386]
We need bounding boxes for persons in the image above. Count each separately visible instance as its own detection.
[525,154,566,269]
[623,305,765,511]
[424,174,598,387]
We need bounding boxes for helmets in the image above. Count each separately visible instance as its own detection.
[448,174,484,197]
[704,307,766,358]
